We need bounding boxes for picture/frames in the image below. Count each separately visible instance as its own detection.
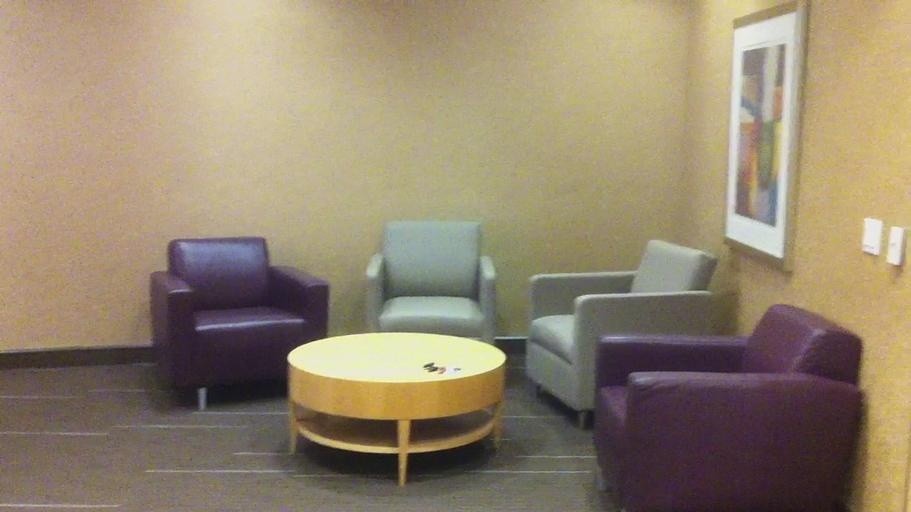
[720,0,810,274]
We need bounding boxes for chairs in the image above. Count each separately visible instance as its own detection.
[525,240,720,430]
[592,303,864,512]
[365,220,497,345]
[150,236,330,411]
